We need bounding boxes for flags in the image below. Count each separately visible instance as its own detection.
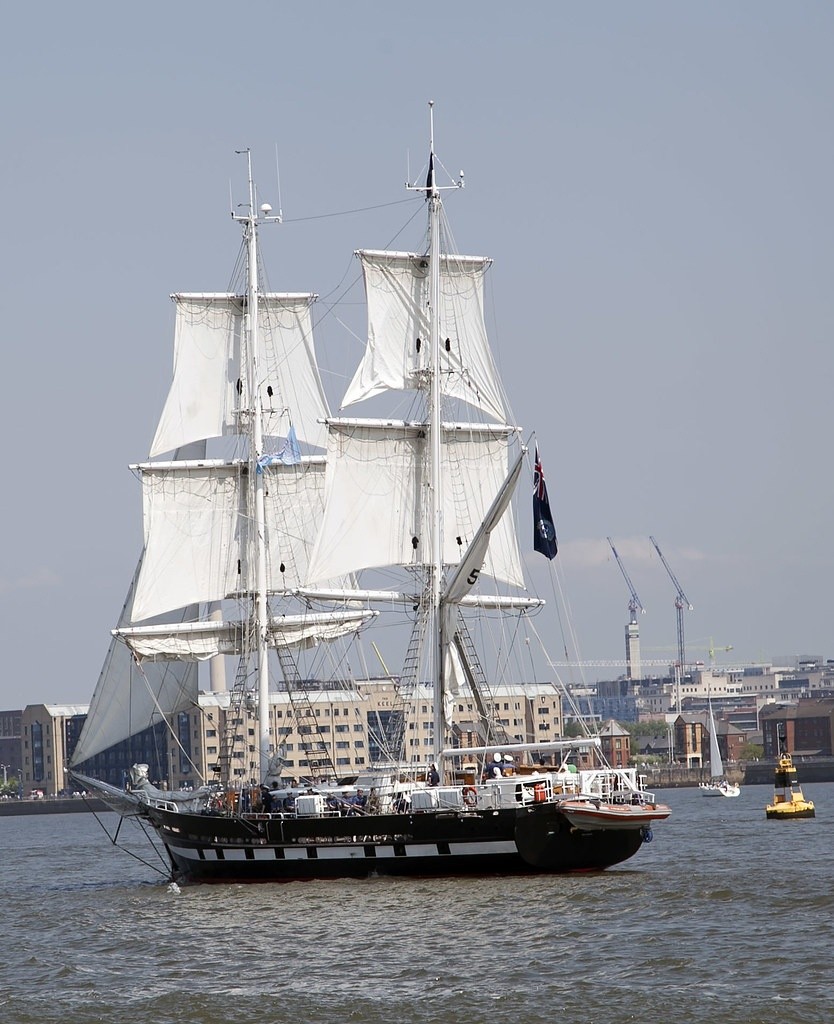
[257,424,300,473]
[533,444,557,562]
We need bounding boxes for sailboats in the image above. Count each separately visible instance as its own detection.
[698,684,741,797]
[62,100,671,878]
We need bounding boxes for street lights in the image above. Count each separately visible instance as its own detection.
[4,764,11,784]
[776,723,783,756]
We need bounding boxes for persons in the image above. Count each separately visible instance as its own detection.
[237,762,440,817]
[718,780,729,791]
[485,752,517,778]
[0,790,93,802]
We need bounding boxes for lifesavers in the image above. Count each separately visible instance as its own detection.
[463,786,479,806]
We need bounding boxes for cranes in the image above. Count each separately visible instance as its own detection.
[546,660,704,714]
[605,535,646,623]
[639,635,735,667]
[648,535,694,682]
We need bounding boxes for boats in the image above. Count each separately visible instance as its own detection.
[766,750,818,819]
[556,797,673,831]
